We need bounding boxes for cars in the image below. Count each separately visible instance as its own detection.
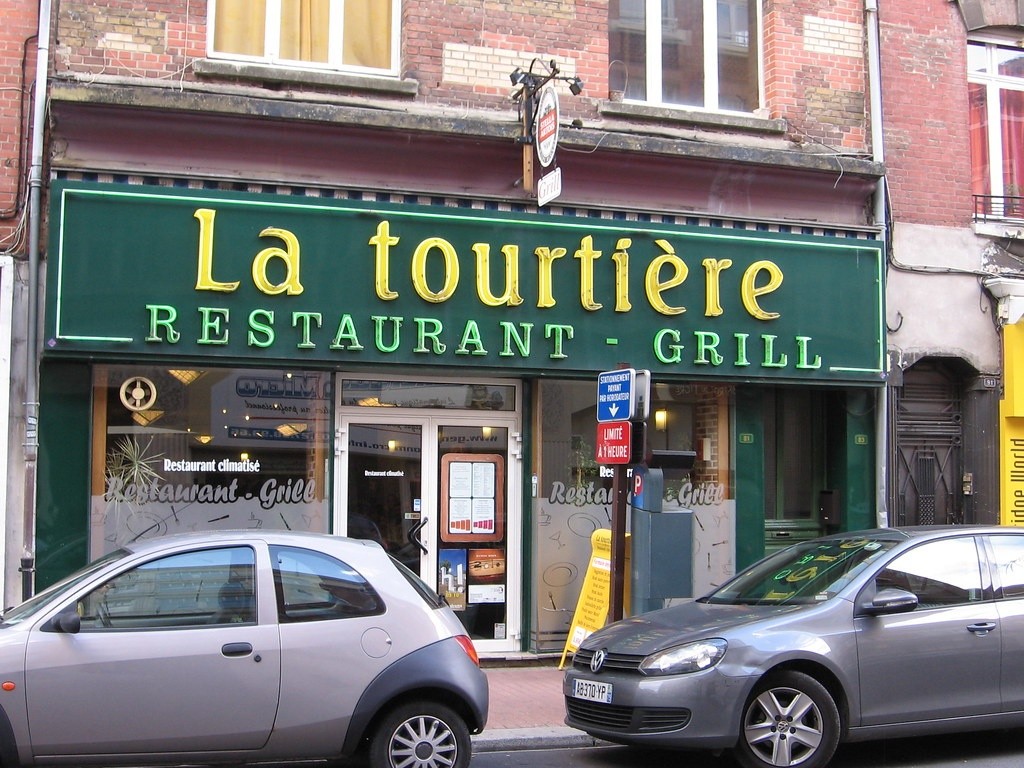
[567,524,1023,767]
[0,527,491,768]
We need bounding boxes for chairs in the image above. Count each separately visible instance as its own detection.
[207,582,246,624]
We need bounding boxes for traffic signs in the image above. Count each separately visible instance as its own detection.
[596,368,635,420]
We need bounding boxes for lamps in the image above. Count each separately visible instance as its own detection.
[510,68,550,86]
[655,408,667,432]
[274,423,308,437]
[131,411,165,427]
[167,369,208,385]
[552,76,583,96]
